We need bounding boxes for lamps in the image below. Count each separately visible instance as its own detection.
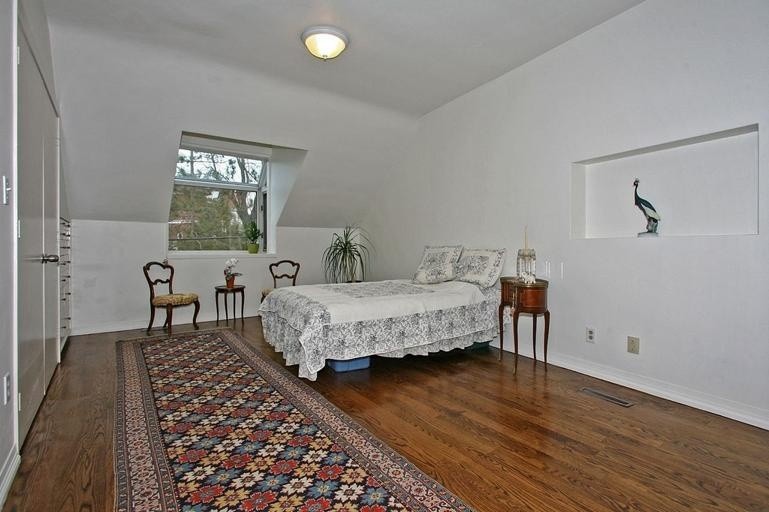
[296,23,352,64]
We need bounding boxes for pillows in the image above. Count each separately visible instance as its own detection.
[409,261,458,287]
[416,243,464,269]
[455,245,507,290]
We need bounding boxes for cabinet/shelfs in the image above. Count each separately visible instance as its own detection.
[19,53,60,457]
[59,213,75,365]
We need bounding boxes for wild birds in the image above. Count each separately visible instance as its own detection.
[633,180,661,233]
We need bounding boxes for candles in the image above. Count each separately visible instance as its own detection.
[523,232,531,260]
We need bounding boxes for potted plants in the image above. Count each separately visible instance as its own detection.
[319,219,378,284]
[244,220,267,255]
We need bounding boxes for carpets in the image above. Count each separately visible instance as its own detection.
[109,324,476,512]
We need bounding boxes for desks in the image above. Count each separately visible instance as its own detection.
[494,275,554,376]
[212,282,249,332]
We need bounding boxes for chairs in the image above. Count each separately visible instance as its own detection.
[140,257,203,339]
[258,260,300,305]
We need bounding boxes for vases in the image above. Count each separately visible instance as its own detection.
[224,275,237,288]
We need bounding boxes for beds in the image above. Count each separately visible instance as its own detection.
[256,278,512,384]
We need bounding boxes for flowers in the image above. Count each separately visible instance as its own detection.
[221,257,244,280]
